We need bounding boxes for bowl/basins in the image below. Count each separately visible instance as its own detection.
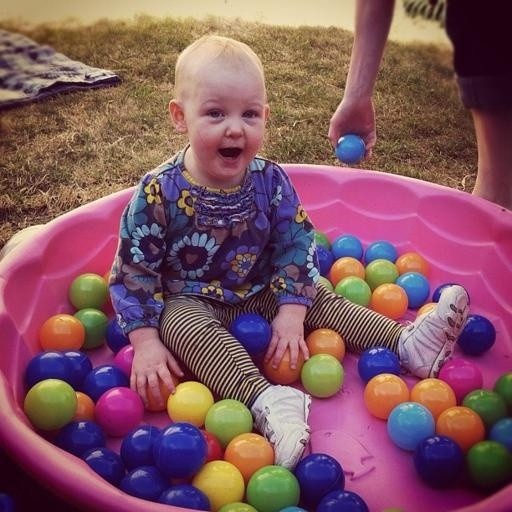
[1,161,511,512]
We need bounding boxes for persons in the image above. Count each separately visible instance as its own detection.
[110,35,469,471]
[327,1,511,209]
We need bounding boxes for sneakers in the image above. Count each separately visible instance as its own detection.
[396,282,472,379]
[250,383,312,471]
